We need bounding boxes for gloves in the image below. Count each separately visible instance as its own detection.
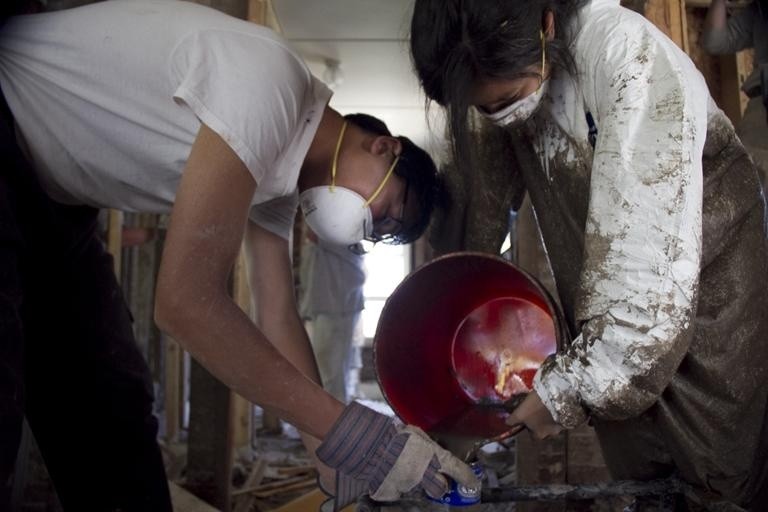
[314,400,479,503]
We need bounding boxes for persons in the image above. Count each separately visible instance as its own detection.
[407,0,766,512]
[699,0,767,194]
[297,208,368,411]
[1,0,486,512]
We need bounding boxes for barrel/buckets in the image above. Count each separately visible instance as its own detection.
[375,253,566,456]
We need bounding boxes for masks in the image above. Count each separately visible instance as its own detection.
[475,43,551,129]
[298,158,399,248]
